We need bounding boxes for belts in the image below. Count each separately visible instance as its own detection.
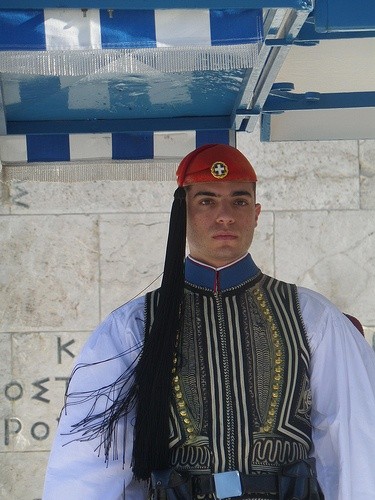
[193,469,280,499]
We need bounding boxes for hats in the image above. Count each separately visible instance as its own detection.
[58,143,257,484]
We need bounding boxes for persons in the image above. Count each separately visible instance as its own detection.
[42,142,375,500]
